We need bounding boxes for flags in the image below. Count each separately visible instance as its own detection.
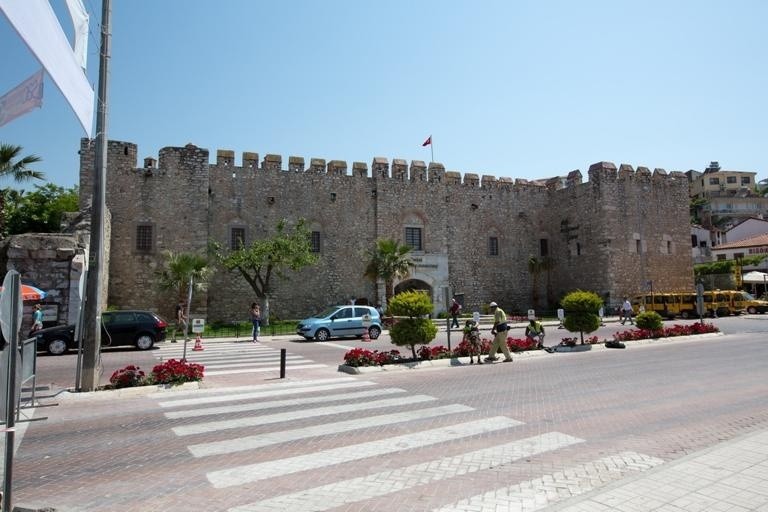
[422,136,431,147]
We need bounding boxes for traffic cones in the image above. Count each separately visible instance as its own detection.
[190,331,205,351]
[361,325,371,342]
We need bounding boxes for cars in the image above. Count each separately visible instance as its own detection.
[296,304,384,343]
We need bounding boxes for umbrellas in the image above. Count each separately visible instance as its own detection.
[0,284,48,302]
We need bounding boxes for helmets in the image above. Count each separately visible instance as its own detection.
[489,301,498,308]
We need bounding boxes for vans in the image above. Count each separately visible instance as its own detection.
[29,308,169,354]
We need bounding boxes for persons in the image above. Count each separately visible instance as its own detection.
[170,299,191,344]
[249,302,262,343]
[30,303,44,334]
[485,301,513,362]
[448,298,460,328]
[524,316,546,347]
[621,296,634,325]
[462,319,485,364]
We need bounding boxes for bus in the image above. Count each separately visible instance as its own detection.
[628,288,767,321]
